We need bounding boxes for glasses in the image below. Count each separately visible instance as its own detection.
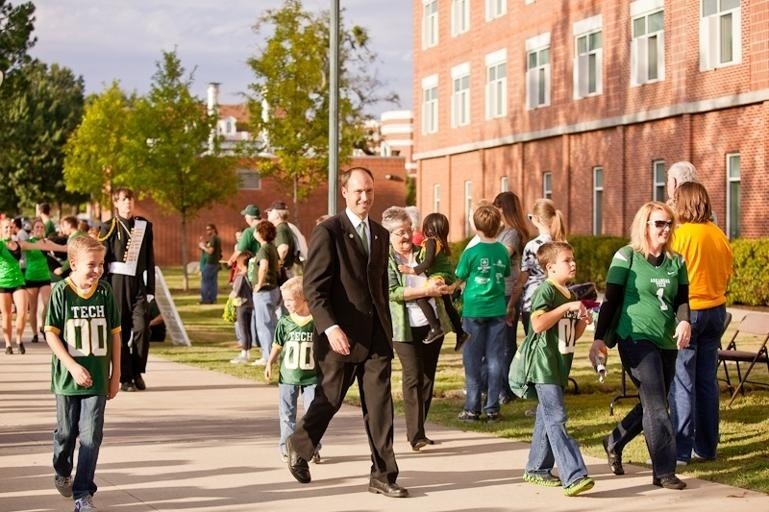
[648,220,672,227]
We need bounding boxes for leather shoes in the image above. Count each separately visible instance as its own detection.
[369,478,408,497]
[286,437,310,483]
[121,374,145,392]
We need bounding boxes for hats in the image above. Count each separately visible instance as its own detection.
[241,205,259,216]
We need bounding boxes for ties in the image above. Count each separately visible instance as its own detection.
[359,223,368,255]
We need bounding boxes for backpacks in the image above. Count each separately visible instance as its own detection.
[508,337,537,399]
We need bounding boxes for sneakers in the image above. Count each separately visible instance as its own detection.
[455,332,469,351]
[6,330,47,353]
[458,410,503,422]
[55,472,72,497]
[523,473,594,496]
[422,327,444,344]
[603,439,625,475]
[654,474,686,489]
[75,495,96,512]
[413,438,434,450]
[231,353,269,365]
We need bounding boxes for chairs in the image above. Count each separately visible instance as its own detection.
[709,310,735,400]
[718,313,769,406]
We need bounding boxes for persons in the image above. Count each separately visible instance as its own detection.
[663,161,718,228]
[0,186,166,512]
[195,167,595,498]
[588,199,693,491]
[664,180,733,466]
